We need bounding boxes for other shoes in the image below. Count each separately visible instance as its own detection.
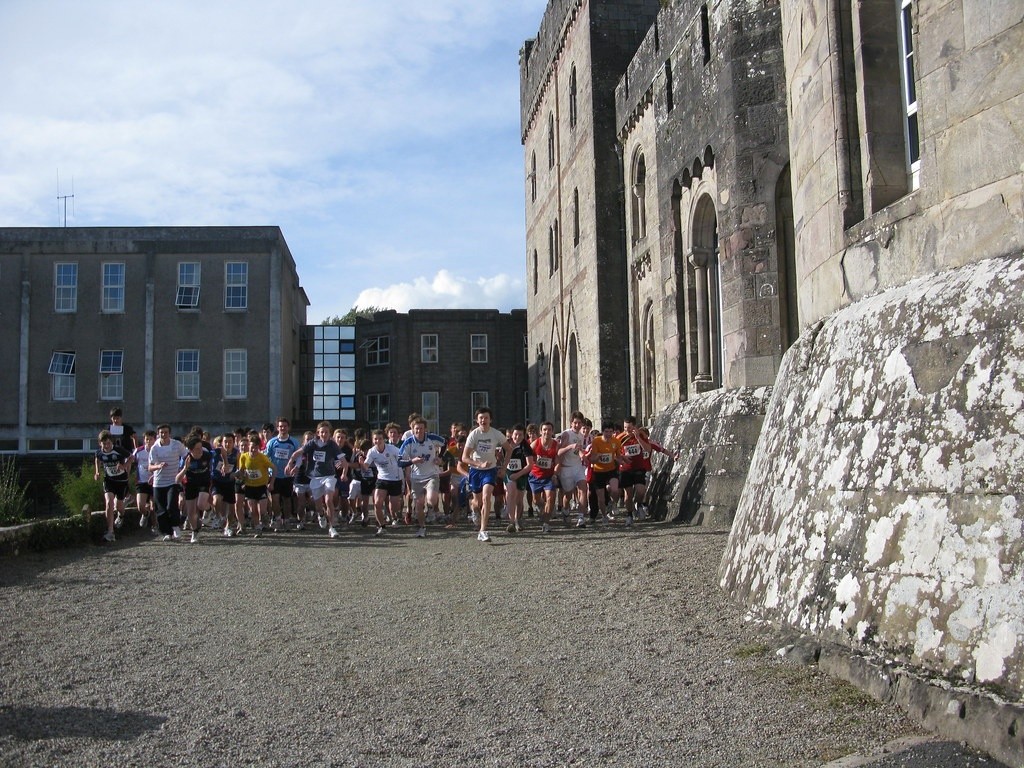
[634,502,645,520]
[625,516,634,527]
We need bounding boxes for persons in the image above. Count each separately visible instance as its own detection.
[94,430,134,541]
[461,407,513,542]
[133,413,678,543]
[108,408,138,510]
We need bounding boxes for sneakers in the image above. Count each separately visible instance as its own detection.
[114,516,124,529]
[417,527,427,538]
[103,532,116,542]
[477,532,490,542]
[535,499,544,511]
[426,509,436,521]
[163,535,171,542]
[506,523,516,533]
[190,532,198,543]
[392,517,399,527]
[563,505,570,514]
[471,507,482,526]
[113,498,118,513]
[375,526,388,535]
[140,510,150,528]
[319,510,325,528]
[124,494,135,508]
[172,526,181,538]
[515,518,523,530]
[152,519,158,537]
[184,501,619,538]
[329,527,340,538]
[543,523,551,531]
[577,517,586,528]
